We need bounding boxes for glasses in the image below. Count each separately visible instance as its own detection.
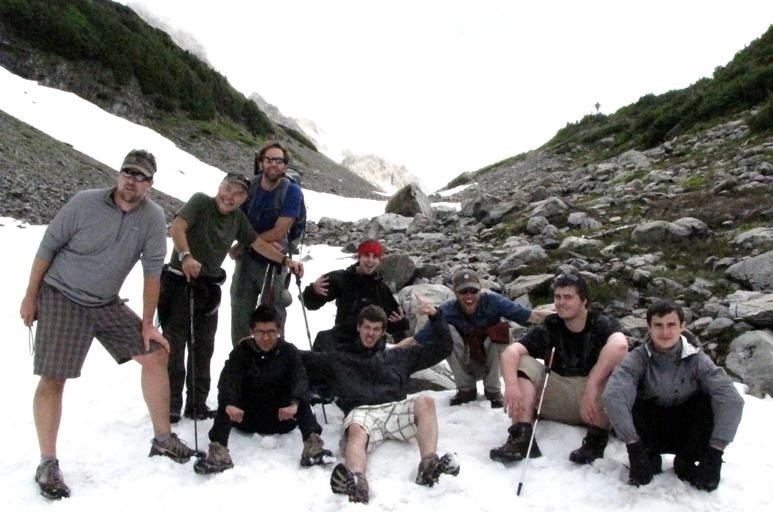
[261,155,286,164]
[556,273,580,282]
[455,287,479,295]
[120,167,151,182]
[251,327,279,337]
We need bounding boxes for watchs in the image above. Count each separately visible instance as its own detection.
[178,249,191,260]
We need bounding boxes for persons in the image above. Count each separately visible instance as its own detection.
[302,292,460,504]
[20,149,206,500]
[156,171,304,422]
[192,303,334,474]
[394,270,556,408]
[489,276,628,465]
[299,240,411,403]
[601,300,744,492]
[231,144,303,346]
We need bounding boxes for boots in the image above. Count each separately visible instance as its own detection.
[569,423,610,464]
[489,422,542,463]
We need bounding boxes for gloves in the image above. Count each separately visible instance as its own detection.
[626,437,653,486]
[689,446,725,493]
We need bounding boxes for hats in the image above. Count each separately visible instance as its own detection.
[358,239,384,257]
[224,172,252,192]
[119,150,157,178]
[453,268,481,291]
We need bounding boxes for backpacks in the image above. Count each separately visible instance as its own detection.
[245,175,306,257]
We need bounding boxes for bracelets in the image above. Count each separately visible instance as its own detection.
[280,254,288,266]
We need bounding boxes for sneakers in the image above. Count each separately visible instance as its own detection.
[194,441,234,474]
[149,433,206,464]
[639,440,662,475]
[169,412,182,423]
[183,405,218,420]
[415,453,461,486]
[34,457,71,500]
[330,462,369,504]
[449,387,477,406]
[670,436,707,482]
[484,388,504,408]
[300,431,334,467]
